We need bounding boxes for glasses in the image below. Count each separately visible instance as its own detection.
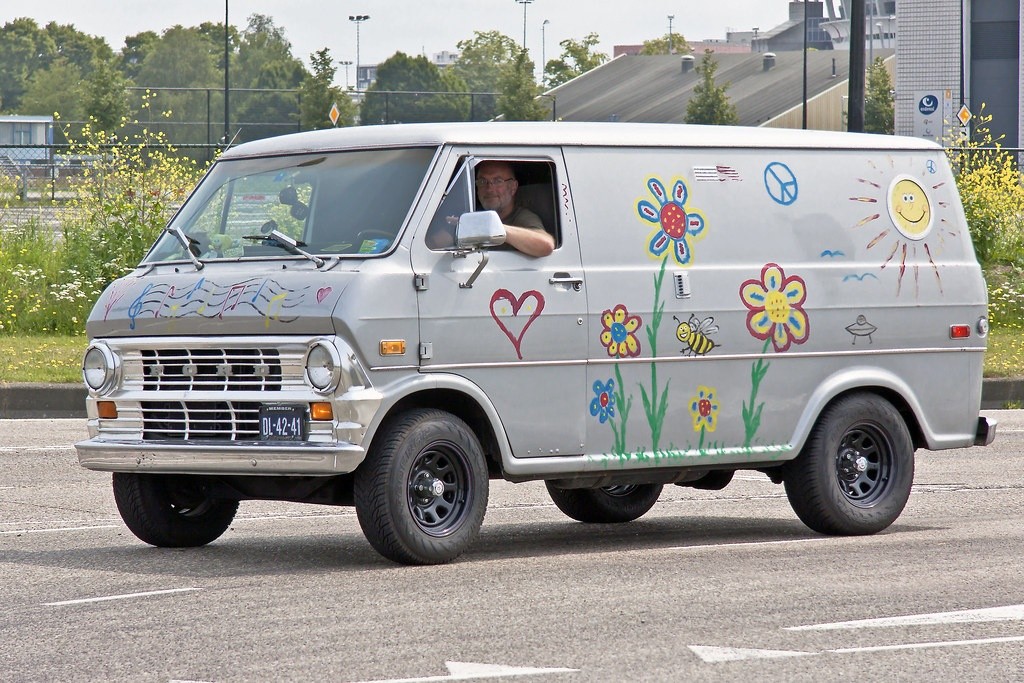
[475,178,513,186]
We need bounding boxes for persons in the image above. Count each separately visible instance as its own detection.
[429,160,555,257]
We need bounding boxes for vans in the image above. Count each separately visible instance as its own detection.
[74,117,1000,571]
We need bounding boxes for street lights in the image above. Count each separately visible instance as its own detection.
[541,19,552,89]
[515,0,535,59]
[349,14,370,114]
[339,60,353,89]
